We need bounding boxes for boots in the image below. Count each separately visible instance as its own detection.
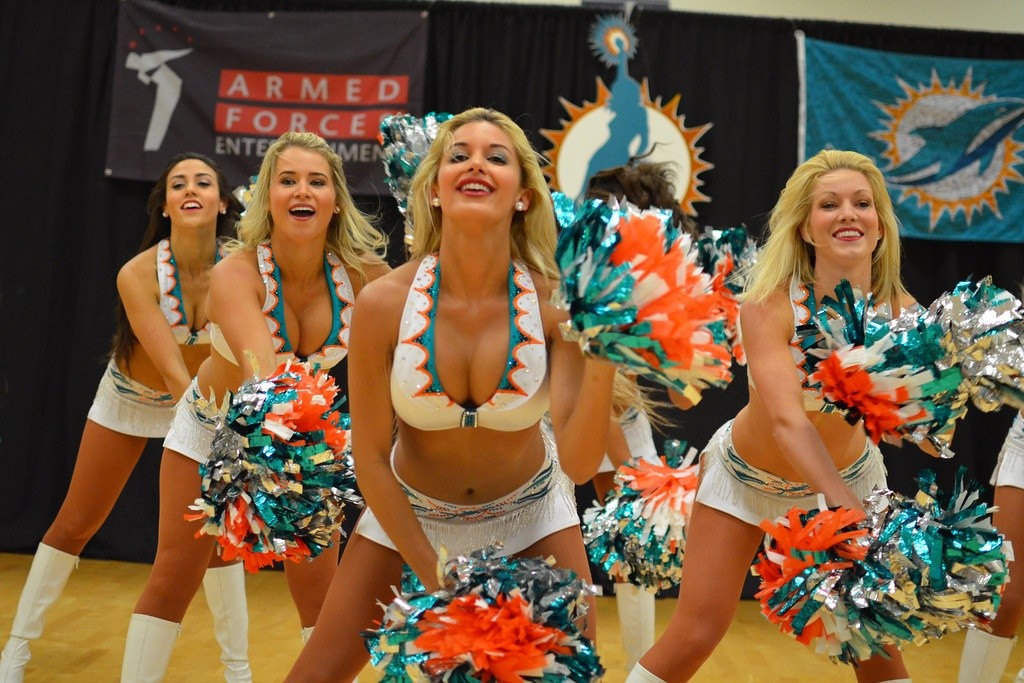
[624,662,662,682]
[0,542,79,683]
[959,623,1015,682]
[202,561,253,682]
[121,614,181,683]
[615,582,655,670]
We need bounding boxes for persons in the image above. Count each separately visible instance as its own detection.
[285,109,617,683]
[0,103,1024,683]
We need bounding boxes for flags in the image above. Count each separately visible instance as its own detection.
[793,30,1024,246]
[103,3,426,197]
